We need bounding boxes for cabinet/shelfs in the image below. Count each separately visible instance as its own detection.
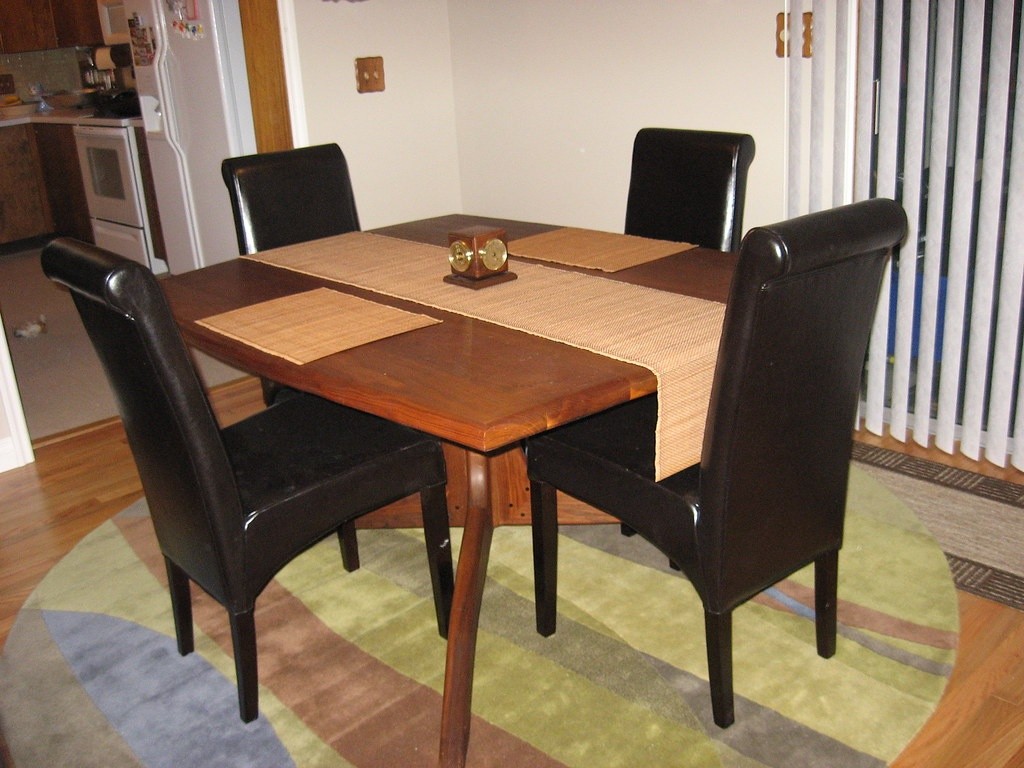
[0,124,48,245]
[0,0,104,53]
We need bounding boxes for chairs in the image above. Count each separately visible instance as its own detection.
[42,236,456,722]
[222,143,361,407]
[521,198,908,730]
[625,129,755,253]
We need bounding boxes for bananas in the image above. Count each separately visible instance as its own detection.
[3,96,24,105]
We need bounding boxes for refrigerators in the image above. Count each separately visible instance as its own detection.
[122,0,257,276]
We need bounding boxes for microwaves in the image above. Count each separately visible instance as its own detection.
[97,0,131,45]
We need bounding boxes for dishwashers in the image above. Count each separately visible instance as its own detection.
[72,126,168,276]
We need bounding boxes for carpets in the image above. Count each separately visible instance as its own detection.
[0,465,959,768]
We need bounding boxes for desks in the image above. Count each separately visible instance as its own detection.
[157,213,737,768]
